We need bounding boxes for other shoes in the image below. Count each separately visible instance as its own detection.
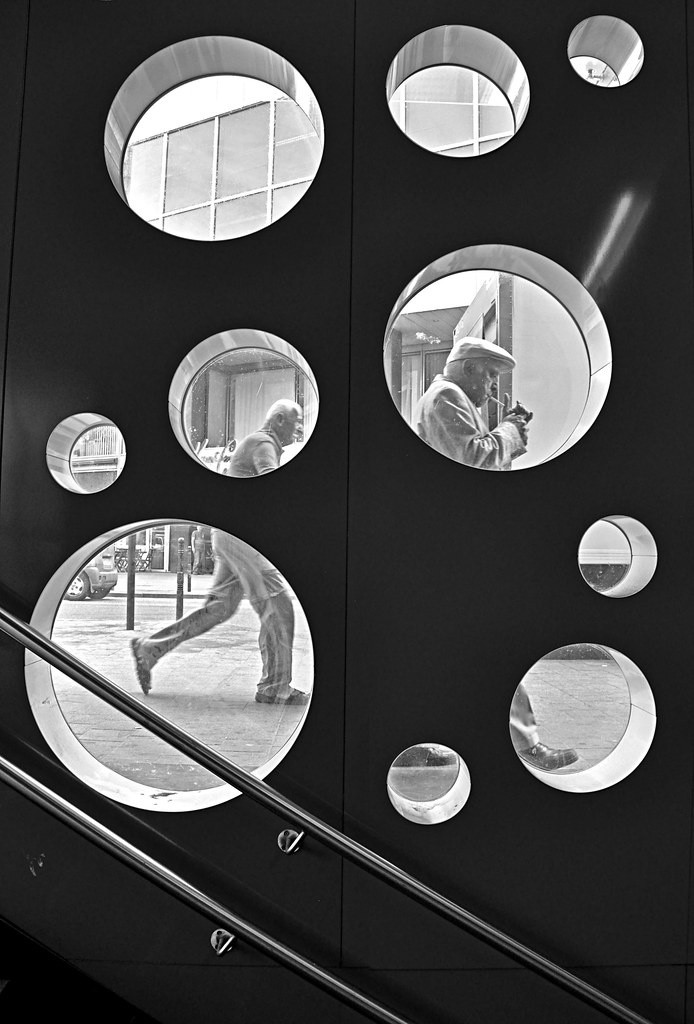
[131,638,152,696]
[255,688,310,705]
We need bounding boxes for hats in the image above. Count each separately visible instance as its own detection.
[446,336,516,375]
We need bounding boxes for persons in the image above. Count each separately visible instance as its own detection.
[124,400,310,705]
[191,525,206,575]
[393,335,578,772]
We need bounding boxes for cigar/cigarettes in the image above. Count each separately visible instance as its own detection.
[490,397,505,406]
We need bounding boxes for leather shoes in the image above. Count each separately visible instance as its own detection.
[520,743,578,771]
[394,747,456,766]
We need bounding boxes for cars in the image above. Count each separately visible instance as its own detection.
[63,541,119,602]
